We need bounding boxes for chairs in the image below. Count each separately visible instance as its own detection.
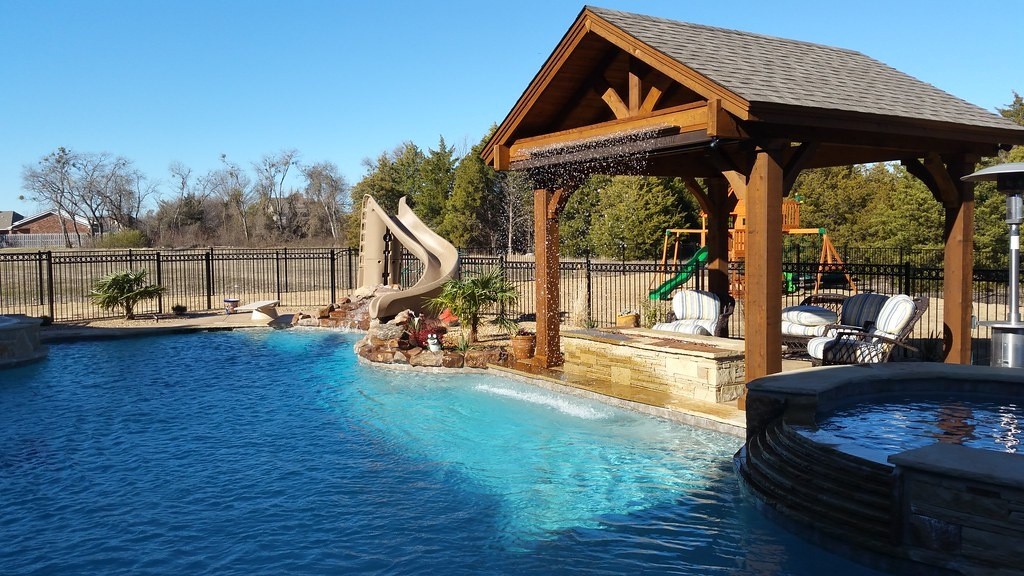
[666,293,735,338]
[812,297,929,369]
[781,293,849,361]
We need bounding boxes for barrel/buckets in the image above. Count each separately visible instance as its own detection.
[224,300,240,315]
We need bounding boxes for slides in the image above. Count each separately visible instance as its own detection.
[367,194,460,320]
[648,245,709,300]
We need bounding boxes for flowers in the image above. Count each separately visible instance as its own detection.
[419,326,448,346]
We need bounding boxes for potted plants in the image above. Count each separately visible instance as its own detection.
[510,327,536,359]
